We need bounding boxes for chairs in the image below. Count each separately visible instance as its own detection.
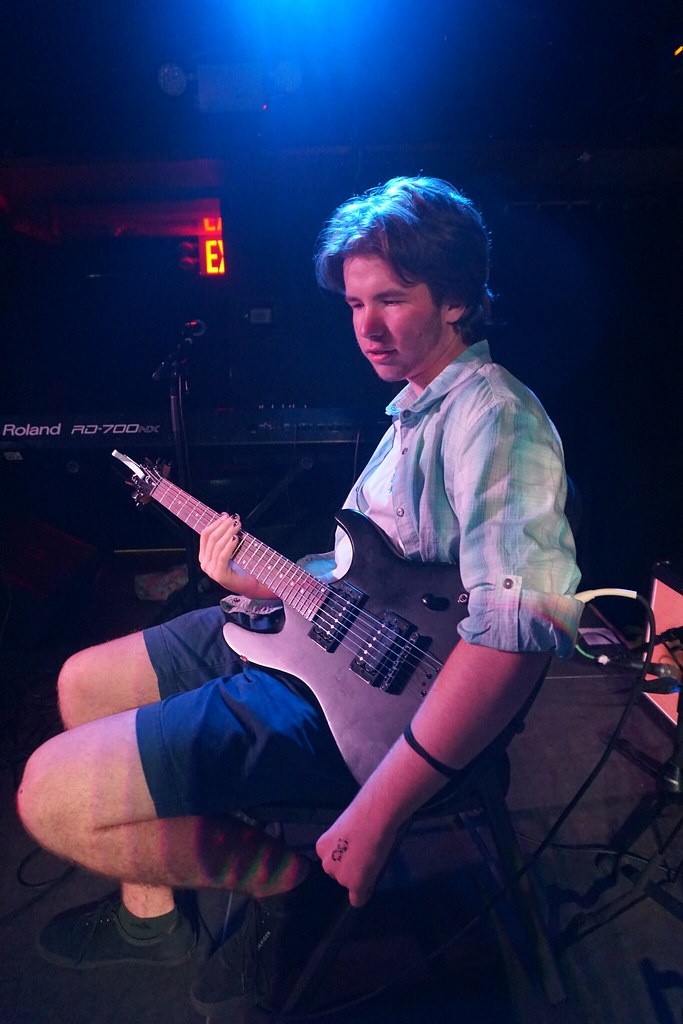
[235,637,569,1022]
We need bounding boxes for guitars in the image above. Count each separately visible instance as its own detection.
[109,446,552,816]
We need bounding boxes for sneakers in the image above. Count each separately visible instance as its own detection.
[34,894,201,971]
[178,844,340,1015]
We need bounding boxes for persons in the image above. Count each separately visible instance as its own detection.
[13,174,587,1020]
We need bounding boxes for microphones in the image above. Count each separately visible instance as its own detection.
[600,654,679,680]
[151,320,208,384]
